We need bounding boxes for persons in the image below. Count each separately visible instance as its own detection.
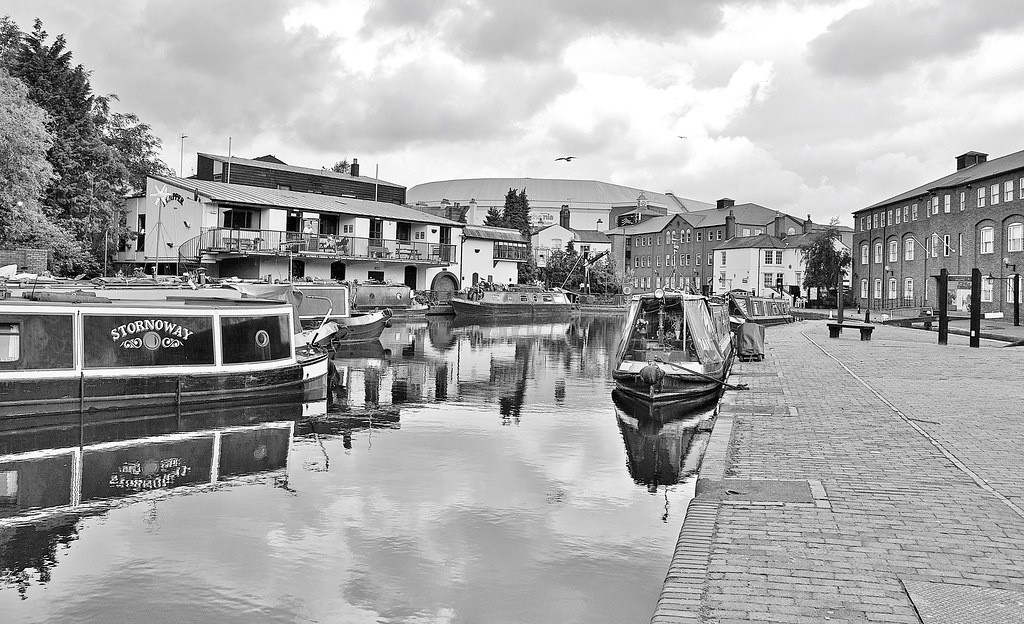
[799,296,810,309]
[339,236,351,256]
[793,296,802,307]
[327,233,336,252]
[301,224,313,251]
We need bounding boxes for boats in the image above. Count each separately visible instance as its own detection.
[216,282,394,344]
[344,282,430,319]
[611,289,736,424]
[451,282,581,313]
[724,291,794,332]
[165,289,347,356]
[0,265,331,423]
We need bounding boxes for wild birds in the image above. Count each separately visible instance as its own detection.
[555,157,576,161]
